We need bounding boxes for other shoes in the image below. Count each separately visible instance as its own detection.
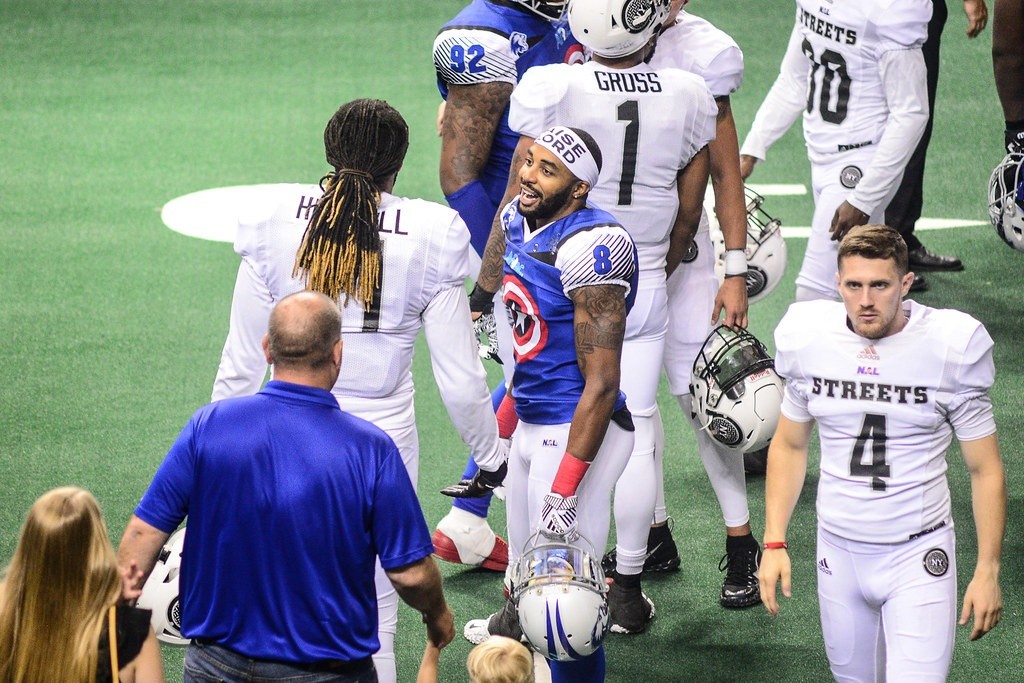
[743,446,769,475]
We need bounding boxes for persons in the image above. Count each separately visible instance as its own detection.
[757,222,1003,683]
[415,634,534,683]
[466,127,636,683]
[991,0,1024,158]
[119,290,456,683]
[734,0,932,309]
[431,0,768,644]
[884,1,988,294]
[214,97,510,683]
[2,484,167,683]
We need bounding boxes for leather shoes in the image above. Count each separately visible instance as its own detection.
[907,275,928,290]
[907,245,963,272]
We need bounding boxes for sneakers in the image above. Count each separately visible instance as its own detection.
[607,581,655,633]
[721,540,762,607]
[463,595,534,654]
[590,516,681,578]
[431,514,509,571]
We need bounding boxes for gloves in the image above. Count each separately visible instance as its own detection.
[1004,129,1024,163]
[540,492,580,543]
[439,460,508,498]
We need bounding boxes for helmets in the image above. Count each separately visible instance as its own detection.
[137,527,191,646]
[988,154,1024,252]
[567,0,672,60]
[689,324,785,456]
[508,528,611,663]
[700,181,787,306]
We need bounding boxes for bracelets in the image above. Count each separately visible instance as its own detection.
[723,247,749,277]
[763,542,789,550]
